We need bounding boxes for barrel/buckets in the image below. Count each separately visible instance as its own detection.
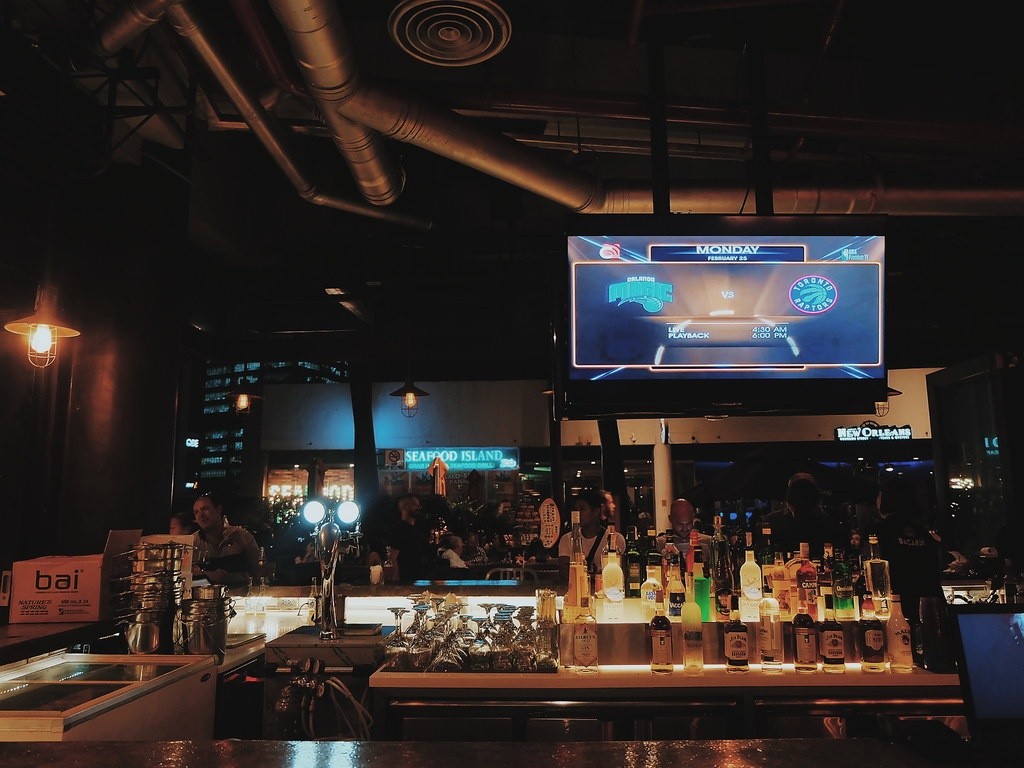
[111,542,236,665]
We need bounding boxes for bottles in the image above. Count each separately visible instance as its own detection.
[255,546,267,585]
[723,594,750,674]
[245,576,265,614]
[857,591,886,673]
[885,595,913,673]
[820,594,846,673]
[912,596,933,669]
[758,575,785,674]
[681,571,704,677]
[601,516,890,619]
[309,577,319,615]
[572,597,598,675]
[793,589,818,674]
[568,511,590,606]
[649,589,674,675]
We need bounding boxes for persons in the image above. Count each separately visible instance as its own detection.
[421,524,548,576]
[342,540,367,564]
[389,494,442,584]
[656,499,713,561]
[192,491,267,587]
[867,479,937,619]
[285,537,315,568]
[558,489,627,572]
[729,472,847,562]
[169,512,196,535]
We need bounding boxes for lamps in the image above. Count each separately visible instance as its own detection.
[875,387,903,416]
[390,373,430,418]
[3,281,83,368]
[227,378,266,414]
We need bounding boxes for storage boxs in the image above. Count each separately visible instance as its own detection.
[9,528,143,623]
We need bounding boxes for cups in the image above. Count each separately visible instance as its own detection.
[370,571,384,589]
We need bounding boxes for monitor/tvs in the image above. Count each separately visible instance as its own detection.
[945,604,1024,725]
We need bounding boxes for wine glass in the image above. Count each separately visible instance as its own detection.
[386,595,555,670]
